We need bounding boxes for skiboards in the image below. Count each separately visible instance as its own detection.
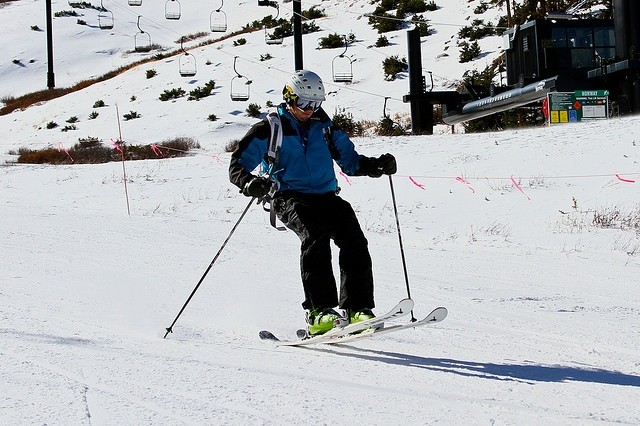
[260,298,447,345]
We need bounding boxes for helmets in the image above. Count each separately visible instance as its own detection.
[285,69,327,102]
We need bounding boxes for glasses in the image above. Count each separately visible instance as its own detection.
[287,98,322,111]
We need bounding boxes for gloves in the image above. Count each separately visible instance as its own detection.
[243,176,271,198]
[375,152,397,176]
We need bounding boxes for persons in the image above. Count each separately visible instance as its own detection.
[227,69,397,337]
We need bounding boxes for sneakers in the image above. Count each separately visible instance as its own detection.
[307,306,349,335]
[345,308,378,333]
